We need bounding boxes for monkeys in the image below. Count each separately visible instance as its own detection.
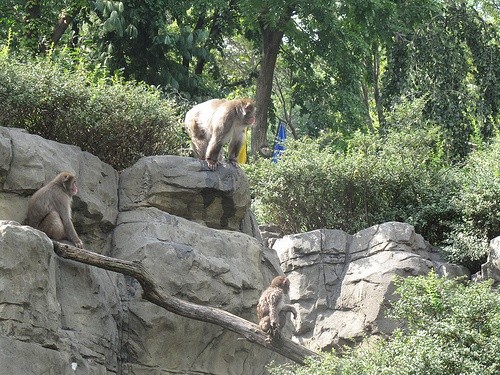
[184,98,257,171]
[21,172,84,250]
[257,276,298,334]
